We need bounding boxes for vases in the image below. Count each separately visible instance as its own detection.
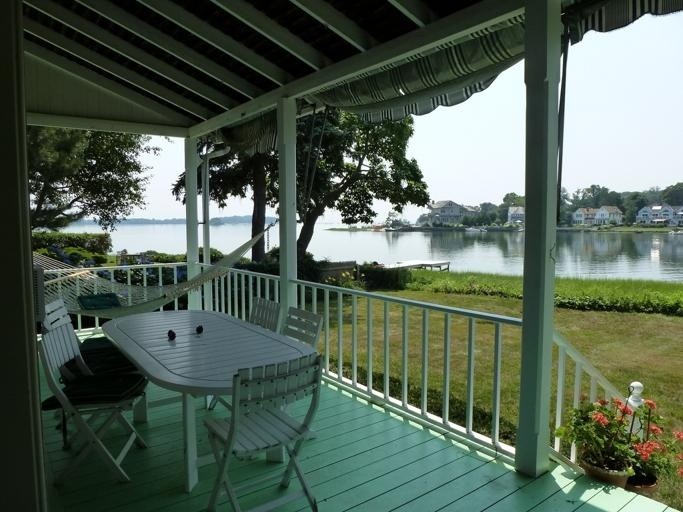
[577,452,633,489]
[630,464,657,487]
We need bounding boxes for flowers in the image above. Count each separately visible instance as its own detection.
[634,399,683,477]
[554,397,642,470]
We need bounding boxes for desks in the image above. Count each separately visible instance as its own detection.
[101,310,319,493]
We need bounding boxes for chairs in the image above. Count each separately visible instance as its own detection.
[41,297,88,353]
[37,322,149,482]
[283,306,322,349]
[203,351,323,512]
[208,296,279,413]
